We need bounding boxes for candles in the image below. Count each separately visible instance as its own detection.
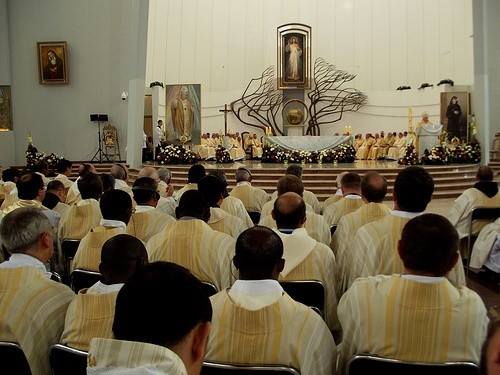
[345,125,351,134]
[265,126,271,134]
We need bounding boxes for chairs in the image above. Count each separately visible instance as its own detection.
[0,207,500,375]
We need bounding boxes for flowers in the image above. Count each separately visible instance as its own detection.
[157,139,201,165]
[399,135,482,166]
[215,145,235,164]
[265,144,356,163]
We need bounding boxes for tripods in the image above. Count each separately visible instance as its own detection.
[91,122,109,163]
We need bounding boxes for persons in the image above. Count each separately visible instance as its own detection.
[148,136,153,143]
[445,95,463,142]
[334,130,417,160]
[0,163,500,375]
[105,132,115,147]
[0,206,78,375]
[285,36,303,80]
[172,87,196,142]
[83,262,212,375]
[339,213,489,375]
[451,167,500,262]
[199,132,263,161]
[142,129,147,164]
[155,120,164,146]
[203,225,337,375]
[44,49,63,78]
[421,109,433,127]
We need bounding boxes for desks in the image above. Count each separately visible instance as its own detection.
[261,136,354,162]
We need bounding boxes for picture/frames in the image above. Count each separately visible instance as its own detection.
[36,40,69,85]
